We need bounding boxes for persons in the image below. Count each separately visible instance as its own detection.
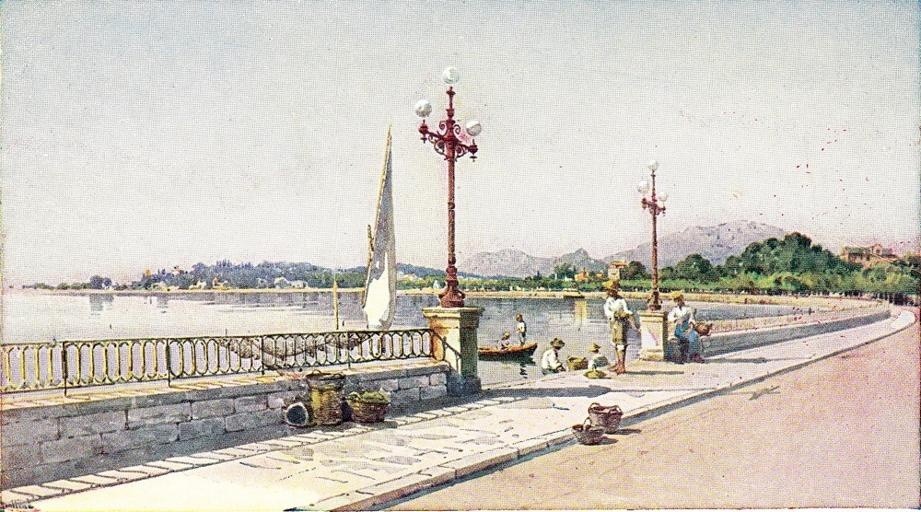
[515,313,527,347]
[497,331,513,352]
[601,280,620,374]
[589,342,609,367]
[604,281,633,374]
[668,289,706,364]
[540,337,566,375]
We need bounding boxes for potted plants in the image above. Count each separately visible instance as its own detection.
[349,390,391,422]
